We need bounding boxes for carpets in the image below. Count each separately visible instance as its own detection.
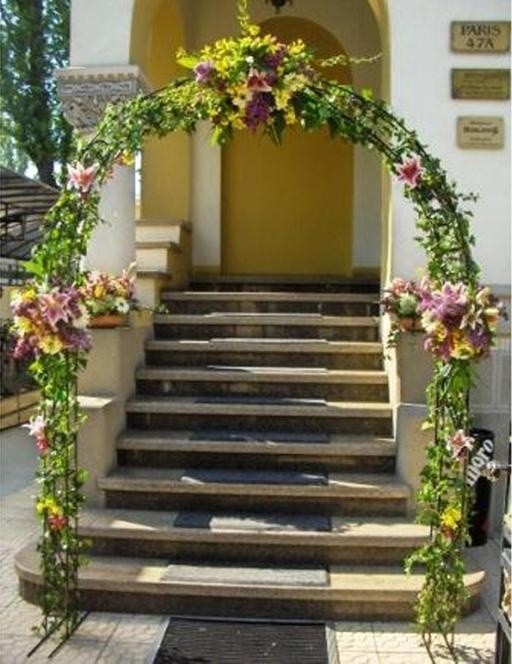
[151,362,342,664]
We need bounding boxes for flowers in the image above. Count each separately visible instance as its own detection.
[20,417,53,450]
[6,262,144,357]
[391,155,425,189]
[367,274,506,364]
[65,162,98,193]
[447,430,474,461]
[173,1,386,145]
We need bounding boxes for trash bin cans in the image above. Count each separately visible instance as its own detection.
[465,428,494,547]
